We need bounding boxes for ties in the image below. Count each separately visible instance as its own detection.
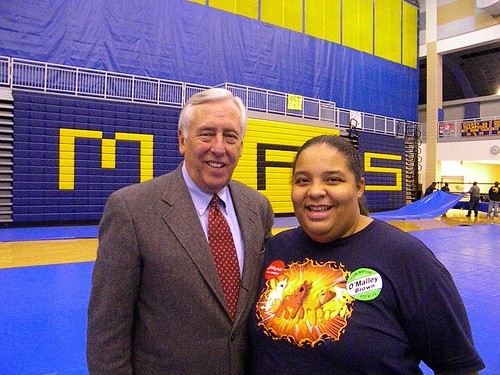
[207,194,241,322]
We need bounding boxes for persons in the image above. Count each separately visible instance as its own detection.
[487,181,500,217]
[466,182,480,217]
[244,135,486,375]
[85,89,274,375]
[424,182,437,196]
[440,182,450,216]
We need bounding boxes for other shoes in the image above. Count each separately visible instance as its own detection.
[466,214,471,217]
[474,214,477,217]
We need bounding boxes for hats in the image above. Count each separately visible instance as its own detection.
[494,182,499,185]
